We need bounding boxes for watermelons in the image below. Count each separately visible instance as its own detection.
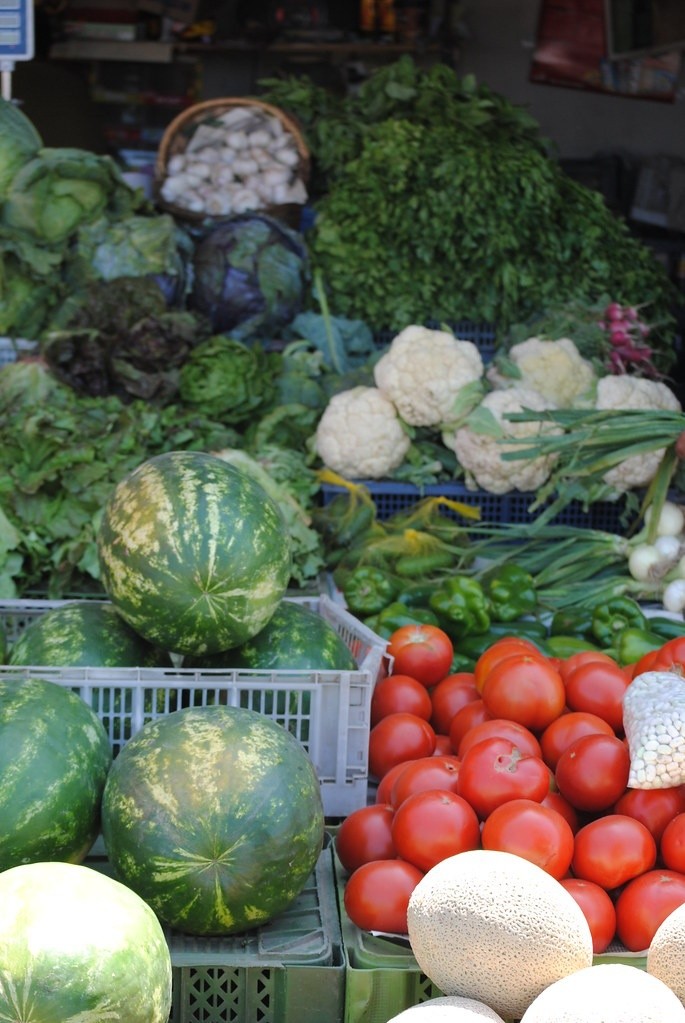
[0,448,359,1021]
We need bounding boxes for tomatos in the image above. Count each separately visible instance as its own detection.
[337,626,685,952]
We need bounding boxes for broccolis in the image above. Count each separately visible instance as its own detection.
[316,323,681,500]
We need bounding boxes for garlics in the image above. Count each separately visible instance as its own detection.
[428,404,685,614]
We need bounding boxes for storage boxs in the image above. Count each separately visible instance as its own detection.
[0,98,683,1020]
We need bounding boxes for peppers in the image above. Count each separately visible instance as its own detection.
[344,564,685,673]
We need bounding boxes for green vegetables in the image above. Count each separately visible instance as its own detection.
[254,52,684,372]
[0,99,328,600]
